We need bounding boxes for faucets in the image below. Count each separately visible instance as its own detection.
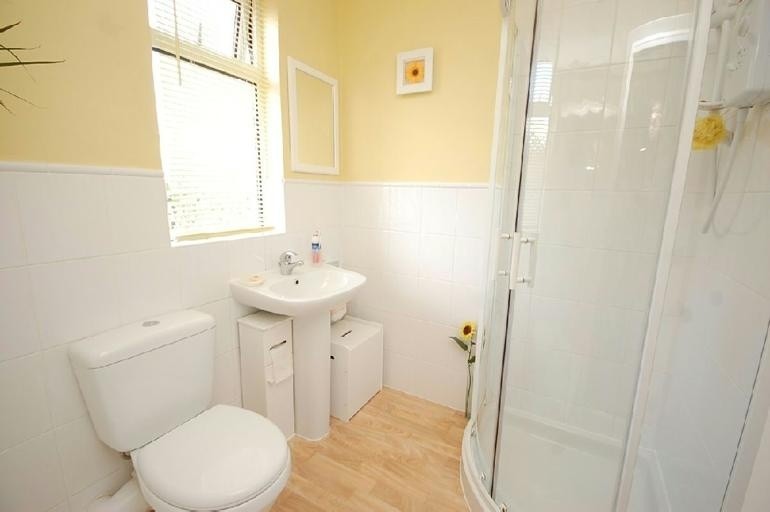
[278,251,305,276]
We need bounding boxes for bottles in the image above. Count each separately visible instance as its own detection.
[311,231,320,265]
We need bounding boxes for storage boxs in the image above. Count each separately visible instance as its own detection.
[236,313,296,440]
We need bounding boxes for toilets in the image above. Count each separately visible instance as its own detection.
[69,310,291,512]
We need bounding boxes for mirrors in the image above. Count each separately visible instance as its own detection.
[287,57,339,175]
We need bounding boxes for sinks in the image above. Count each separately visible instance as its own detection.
[231,263,367,317]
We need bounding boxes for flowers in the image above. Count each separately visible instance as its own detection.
[448,319,477,418]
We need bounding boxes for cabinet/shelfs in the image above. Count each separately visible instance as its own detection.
[331,315,383,421]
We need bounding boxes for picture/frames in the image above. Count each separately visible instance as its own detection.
[396,47,433,95]
